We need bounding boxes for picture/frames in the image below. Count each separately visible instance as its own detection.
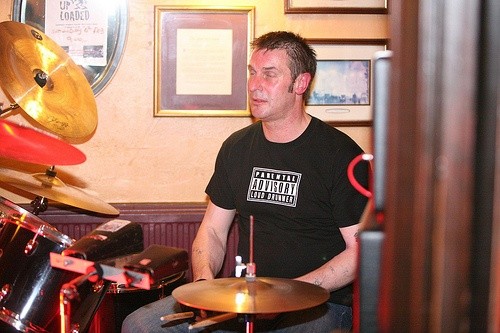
[300,38,391,127]
[284,0,388,15]
[13,0,128,98]
[152,5,257,118]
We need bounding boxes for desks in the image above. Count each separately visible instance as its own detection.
[85,250,185,333]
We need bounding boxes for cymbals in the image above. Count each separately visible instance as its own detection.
[0,160,120,217]
[0,20,99,141]
[171,276,330,314]
[0,121,87,165]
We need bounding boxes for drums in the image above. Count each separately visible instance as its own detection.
[59,255,188,333]
[0,196,58,230]
[0,216,111,333]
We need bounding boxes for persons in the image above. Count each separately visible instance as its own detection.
[121,31,373,333]
